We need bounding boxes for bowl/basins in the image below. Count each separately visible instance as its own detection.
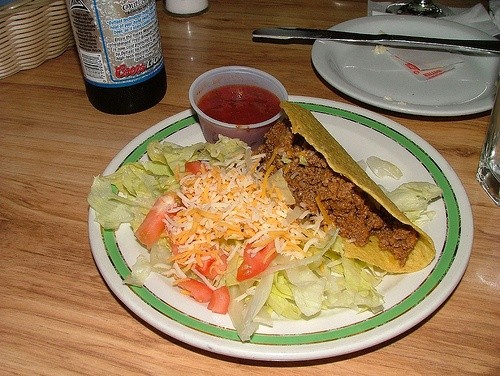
[189,66,288,148]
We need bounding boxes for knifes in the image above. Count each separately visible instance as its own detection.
[253,27,500,56]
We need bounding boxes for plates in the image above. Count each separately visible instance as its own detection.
[88,95,475,360]
[311,16,500,116]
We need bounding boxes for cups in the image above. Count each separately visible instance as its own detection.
[474,78,499,206]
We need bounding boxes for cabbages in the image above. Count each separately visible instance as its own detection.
[87,134,444,343]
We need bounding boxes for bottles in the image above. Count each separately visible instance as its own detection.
[65,0,167,113]
